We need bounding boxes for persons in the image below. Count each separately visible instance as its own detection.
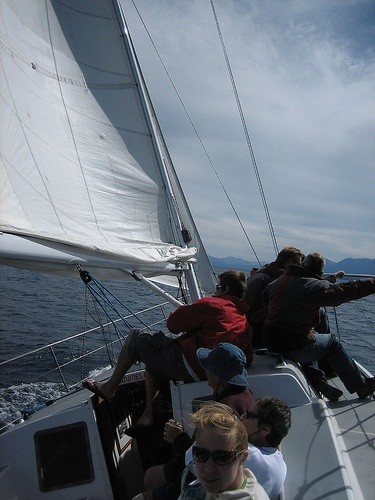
[188,403,270,500]
[244,246,375,401]
[81,271,254,438]
[131,395,292,500]
[144,342,256,492]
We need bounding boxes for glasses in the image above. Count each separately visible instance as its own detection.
[245,408,261,419]
[216,285,222,291]
[191,446,246,466]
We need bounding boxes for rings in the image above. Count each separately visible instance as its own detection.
[164,433,166,437]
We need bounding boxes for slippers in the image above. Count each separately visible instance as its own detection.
[82,380,112,403]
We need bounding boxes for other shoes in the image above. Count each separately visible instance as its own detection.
[357,376,375,399]
[310,371,343,400]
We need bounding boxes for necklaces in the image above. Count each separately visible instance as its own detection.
[261,447,277,453]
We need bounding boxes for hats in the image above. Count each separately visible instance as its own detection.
[196,343,249,387]
